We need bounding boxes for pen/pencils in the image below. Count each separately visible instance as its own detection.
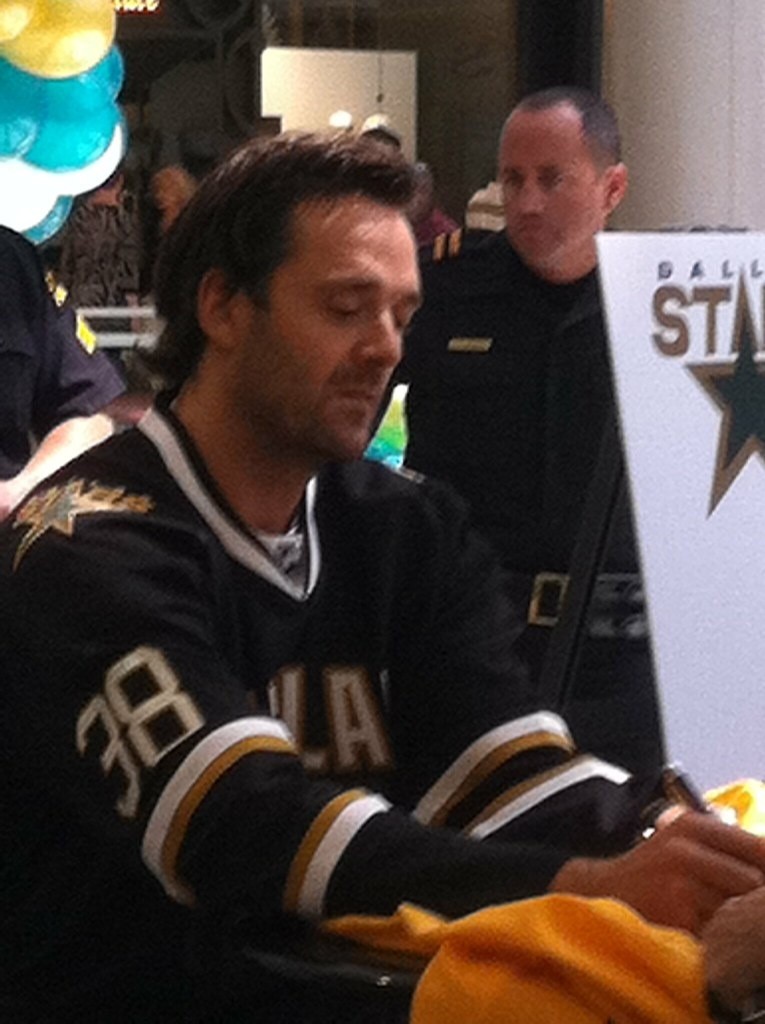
[664,761,714,816]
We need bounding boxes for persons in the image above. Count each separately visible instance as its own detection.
[0,133,765,1024]
[29,161,198,393]
[360,131,457,249]
[402,89,626,674]
[0,226,127,523]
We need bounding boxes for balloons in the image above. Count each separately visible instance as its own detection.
[0,0,126,244]
